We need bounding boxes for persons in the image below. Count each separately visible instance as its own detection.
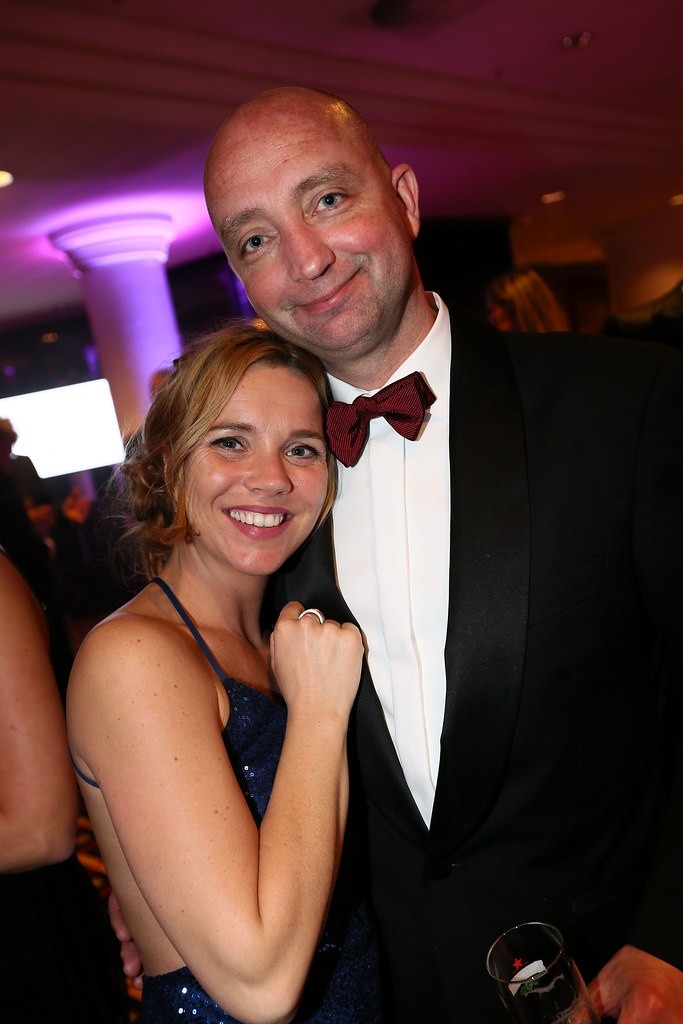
[486,269,569,335]
[64,325,394,1024]
[107,84,683,1024]
[0,421,129,1024]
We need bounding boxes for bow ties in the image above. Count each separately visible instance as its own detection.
[325,371,438,469]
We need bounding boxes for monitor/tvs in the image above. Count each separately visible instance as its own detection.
[0,377,126,480]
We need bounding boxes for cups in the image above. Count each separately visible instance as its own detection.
[486,922,600,1024]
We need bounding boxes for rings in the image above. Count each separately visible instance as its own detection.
[299,609,325,624]
